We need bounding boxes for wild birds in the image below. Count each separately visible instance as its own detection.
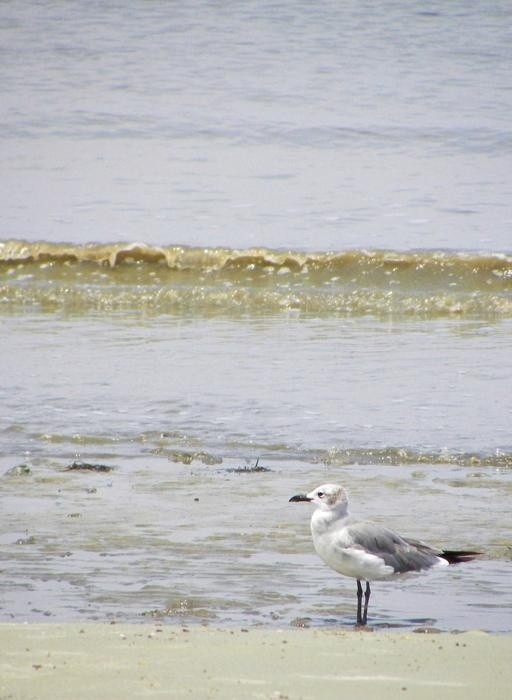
[287,483,485,626]
